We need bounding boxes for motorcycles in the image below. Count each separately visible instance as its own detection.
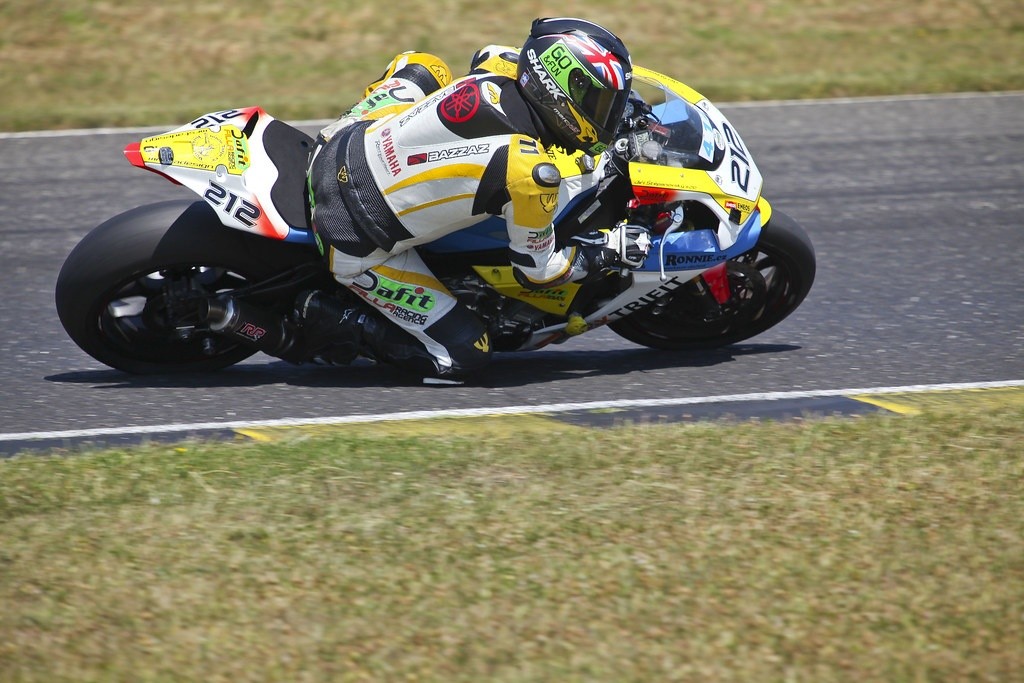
[55,54,815,376]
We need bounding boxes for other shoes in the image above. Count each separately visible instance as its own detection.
[293,294,368,356]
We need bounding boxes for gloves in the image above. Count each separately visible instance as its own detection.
[568,220,652,280]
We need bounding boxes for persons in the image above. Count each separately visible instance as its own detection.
[306,16,653,382]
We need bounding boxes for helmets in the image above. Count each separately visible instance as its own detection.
[515,16,633,157]
